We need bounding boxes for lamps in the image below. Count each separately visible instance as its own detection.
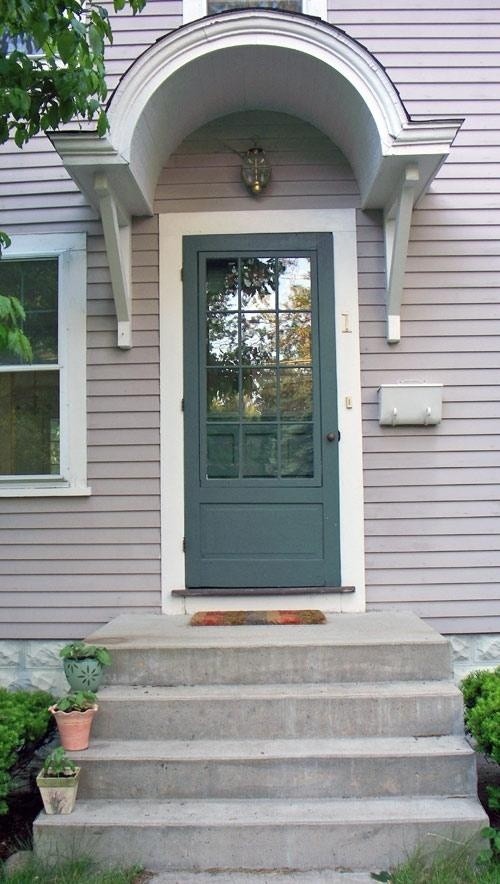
[240,133,272,195]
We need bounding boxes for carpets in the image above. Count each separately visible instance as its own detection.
[189,610,327,626]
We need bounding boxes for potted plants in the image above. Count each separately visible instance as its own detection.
[34,639,111,818]
